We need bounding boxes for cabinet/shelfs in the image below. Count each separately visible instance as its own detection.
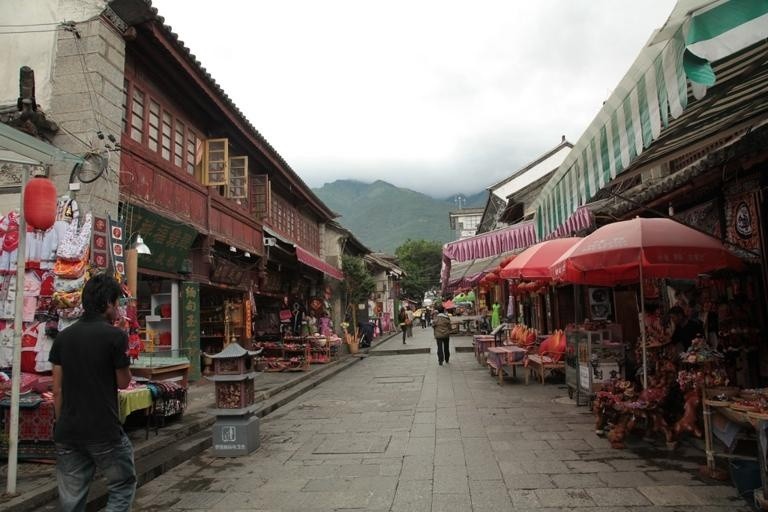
[144,279,178,359]
[567,324,623,411]
[199,320,225,338]
[252,334,332,372]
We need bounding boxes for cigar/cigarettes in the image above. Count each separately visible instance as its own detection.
[121,316,131,321]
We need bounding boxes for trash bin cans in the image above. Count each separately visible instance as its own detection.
[358,322,377,346]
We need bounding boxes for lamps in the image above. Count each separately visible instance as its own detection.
[124,231,152,255]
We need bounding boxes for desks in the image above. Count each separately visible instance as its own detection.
[128,363,192,388]
[472,334,499,366]
[486,345,530,387]
[117,387,159,440]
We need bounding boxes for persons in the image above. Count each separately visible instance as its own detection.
[492,302,501,328]
[432,307,451,365]
[668,306,706,353]
[398,306,433,344]
[320,311,329,336]
[305,310,317,334]
[49,274,137,512]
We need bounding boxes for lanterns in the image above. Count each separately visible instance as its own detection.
[23,175,57,242]
[479,255,548,297]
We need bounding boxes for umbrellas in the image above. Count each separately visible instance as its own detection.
[548,215,744,391]
[500,237,584,405]
[453,291,475,302]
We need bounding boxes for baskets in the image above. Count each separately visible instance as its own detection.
[705,387,740,400]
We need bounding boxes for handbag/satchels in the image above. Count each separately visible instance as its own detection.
[51,214,91,319]
[404,318,411,325]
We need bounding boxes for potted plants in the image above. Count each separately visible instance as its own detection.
[331,250,377,355]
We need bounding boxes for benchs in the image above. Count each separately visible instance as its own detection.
[527,328,566,385]
